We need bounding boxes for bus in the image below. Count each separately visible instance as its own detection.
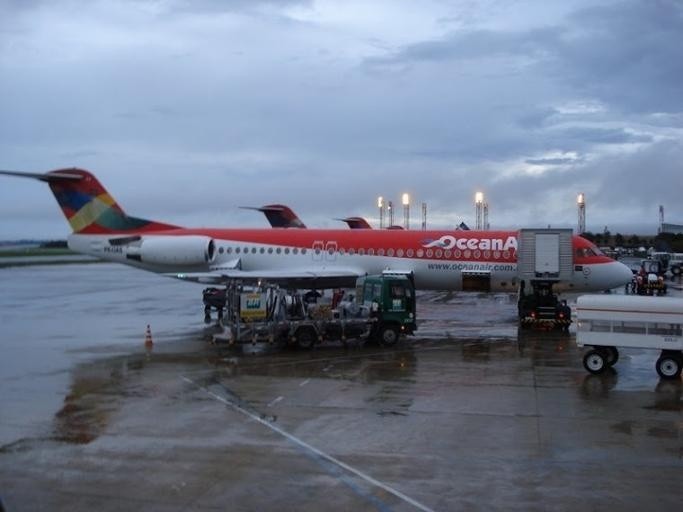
[575,294,683,381]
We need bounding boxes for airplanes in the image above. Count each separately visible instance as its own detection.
[0,165,635,352]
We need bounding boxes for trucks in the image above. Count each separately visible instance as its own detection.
[212,272,417,350]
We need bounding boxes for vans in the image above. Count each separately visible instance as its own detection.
[597,245,682,296]
